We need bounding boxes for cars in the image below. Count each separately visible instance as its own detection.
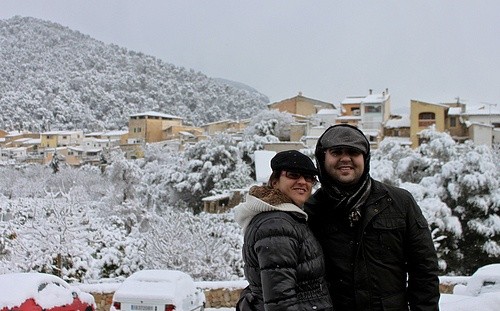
[0,272,97,311]
[110,269,207,311]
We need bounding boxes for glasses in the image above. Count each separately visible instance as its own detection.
[285,168,318,184]
[323,149,361,157]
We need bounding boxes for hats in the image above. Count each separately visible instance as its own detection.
[270,150,320,175]
[320,125,369,155]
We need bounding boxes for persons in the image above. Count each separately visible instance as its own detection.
[236,123,440,311]
[233,149,333,311]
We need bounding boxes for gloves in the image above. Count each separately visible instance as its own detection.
[238,293,255,311]
